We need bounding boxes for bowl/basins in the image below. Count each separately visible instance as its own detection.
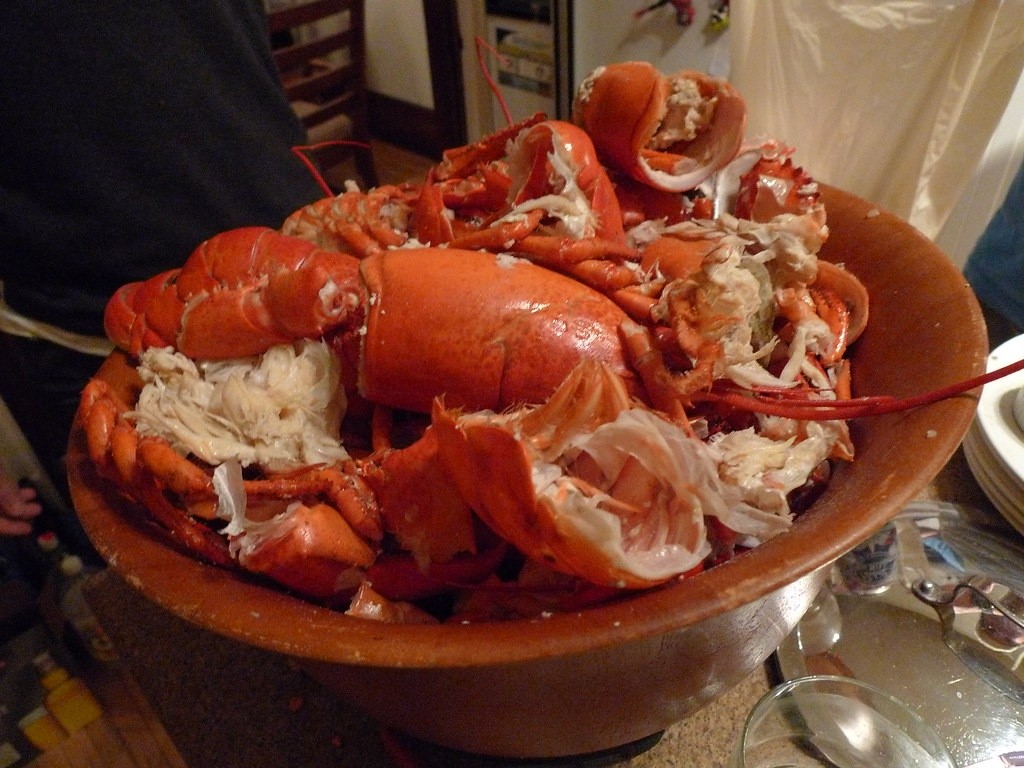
[67,182,987,753]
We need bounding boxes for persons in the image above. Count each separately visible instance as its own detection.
[962,160,1024,354]
[0,1,325,535]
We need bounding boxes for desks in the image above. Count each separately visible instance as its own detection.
[78,449,1024,768]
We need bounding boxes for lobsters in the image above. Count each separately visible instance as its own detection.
[75,35,1024,617]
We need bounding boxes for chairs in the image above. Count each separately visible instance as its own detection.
[266,0,378,189]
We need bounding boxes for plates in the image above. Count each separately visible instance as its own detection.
[963,334,1024,534]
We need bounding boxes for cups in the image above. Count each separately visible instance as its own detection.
[734,676,958,768]
